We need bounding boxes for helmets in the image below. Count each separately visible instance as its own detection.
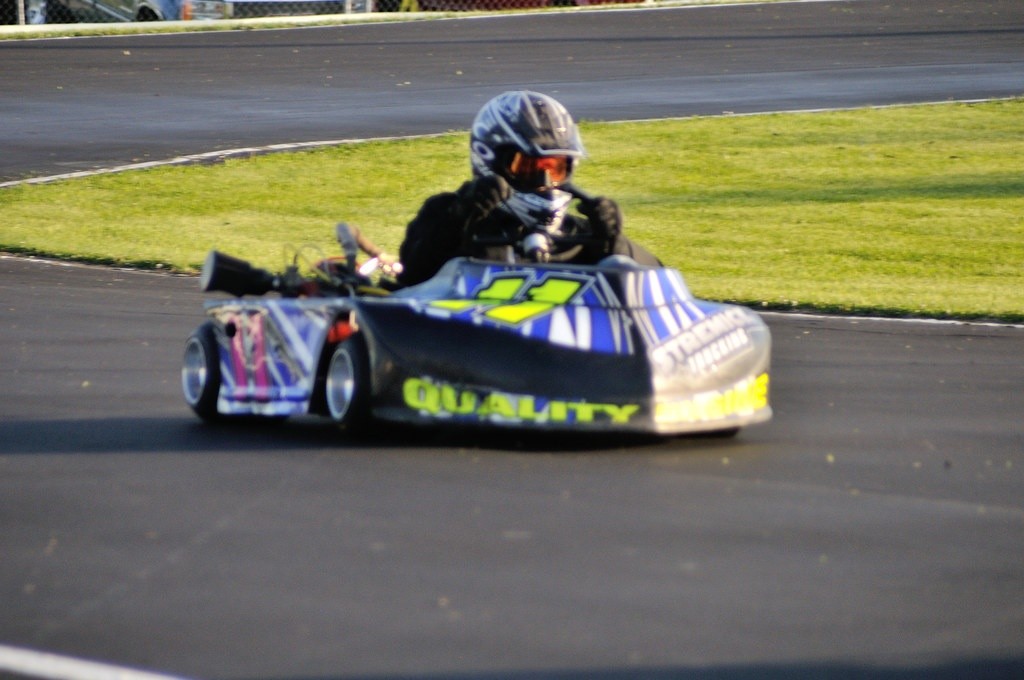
[469,91,584,235]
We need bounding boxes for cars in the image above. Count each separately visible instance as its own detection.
[0,1,378,28]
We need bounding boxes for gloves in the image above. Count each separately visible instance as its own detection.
[577,195,621,254]
[468,209,527,248]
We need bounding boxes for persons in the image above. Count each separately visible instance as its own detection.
[398,91,622,291]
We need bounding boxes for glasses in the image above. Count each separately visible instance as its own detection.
[485,144,574,192]
